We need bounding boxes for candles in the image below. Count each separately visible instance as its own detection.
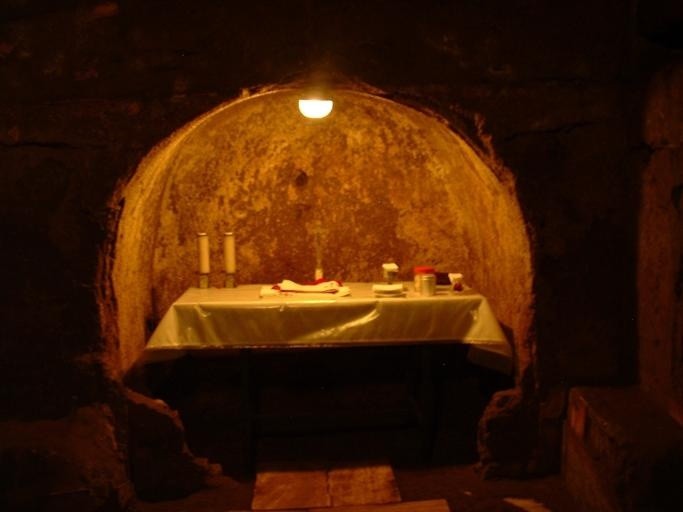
[196,232,210,274]
[223,231,236,274]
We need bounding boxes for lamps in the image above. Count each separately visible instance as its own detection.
[296,60,336,120]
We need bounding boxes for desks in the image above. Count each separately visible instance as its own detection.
[172,282,486,465]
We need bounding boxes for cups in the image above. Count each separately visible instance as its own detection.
[415,274,437,297]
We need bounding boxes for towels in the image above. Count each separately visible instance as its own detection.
[279,278,339,294]
